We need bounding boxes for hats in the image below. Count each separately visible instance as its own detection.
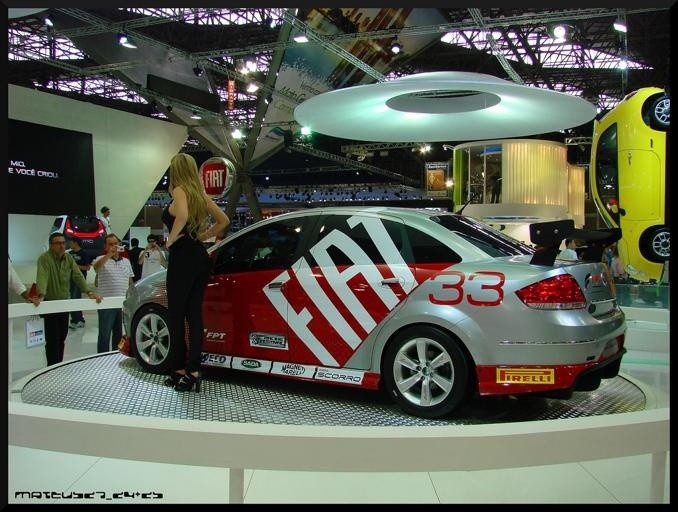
[68,237,82,245]
[101,206,109,212]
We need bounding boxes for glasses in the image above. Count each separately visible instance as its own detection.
[51,241,65,245]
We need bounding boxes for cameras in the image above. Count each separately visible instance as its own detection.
[117,246,124,252]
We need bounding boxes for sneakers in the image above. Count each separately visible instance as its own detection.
[69,320,84,329]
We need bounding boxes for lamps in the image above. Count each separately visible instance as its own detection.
[293,71,597,142]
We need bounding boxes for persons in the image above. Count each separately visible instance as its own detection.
[138,234,167,279]
[33,233,104,367]
[8,259,40,306]
[67,237,92,330]
[92,233,135,353]
[128,238,144,281]
[99,207,112,233]
[159,152,231,393]
[555,238,583,261]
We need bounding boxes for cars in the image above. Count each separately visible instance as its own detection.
[117,206,626,419]
[44,213,107,267]
[589,86,670,284]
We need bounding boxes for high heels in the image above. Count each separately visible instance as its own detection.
[164,372,201,392]
